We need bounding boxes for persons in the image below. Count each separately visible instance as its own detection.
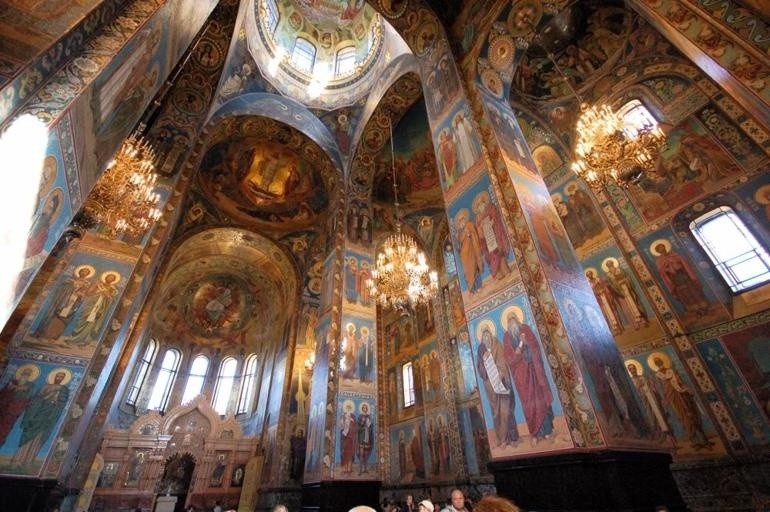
[274,486,518,512]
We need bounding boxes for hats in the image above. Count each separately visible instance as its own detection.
[419,499,434,512]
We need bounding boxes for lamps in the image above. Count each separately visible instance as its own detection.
[534,36,668,193]
[365,109,439,319]
[72,18,213,241]
